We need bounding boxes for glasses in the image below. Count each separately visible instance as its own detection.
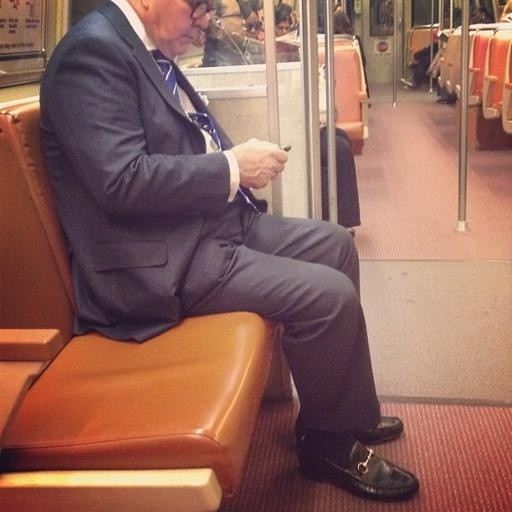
[187,0,219,24]
[215,11,263,32]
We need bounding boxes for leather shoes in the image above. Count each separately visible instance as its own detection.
[296,433,419,502]
[295,410,404,445]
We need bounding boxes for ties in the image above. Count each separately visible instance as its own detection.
[152,49,263,218]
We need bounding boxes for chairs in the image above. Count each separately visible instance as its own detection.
[2,99,280,338]
[5,311,276,511]
[299,33,369,155]
[437,23,512,134]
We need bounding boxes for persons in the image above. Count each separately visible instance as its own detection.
[203,0,360,239]
[239,0,494,107]
[40,0,419,503]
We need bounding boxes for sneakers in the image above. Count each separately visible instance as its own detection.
[437,96,457,104]
[400,77,416,88]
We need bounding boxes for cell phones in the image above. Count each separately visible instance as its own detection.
[279,143,291,153]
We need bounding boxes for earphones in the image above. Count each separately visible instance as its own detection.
[216,20,222,26]
